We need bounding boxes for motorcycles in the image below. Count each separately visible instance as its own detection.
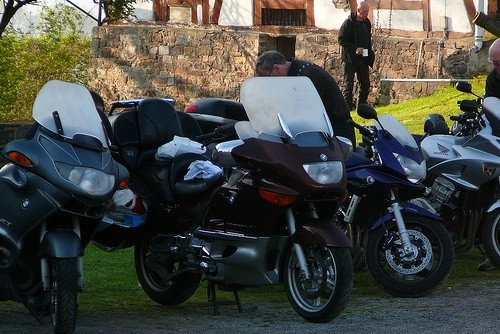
[93,80,500,308]
[0,79,130,334]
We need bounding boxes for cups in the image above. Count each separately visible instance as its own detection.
[361,49,368,56]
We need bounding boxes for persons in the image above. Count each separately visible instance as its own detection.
[256,51,356,151]
[477,37,500,272]
[338,2,375,111]
